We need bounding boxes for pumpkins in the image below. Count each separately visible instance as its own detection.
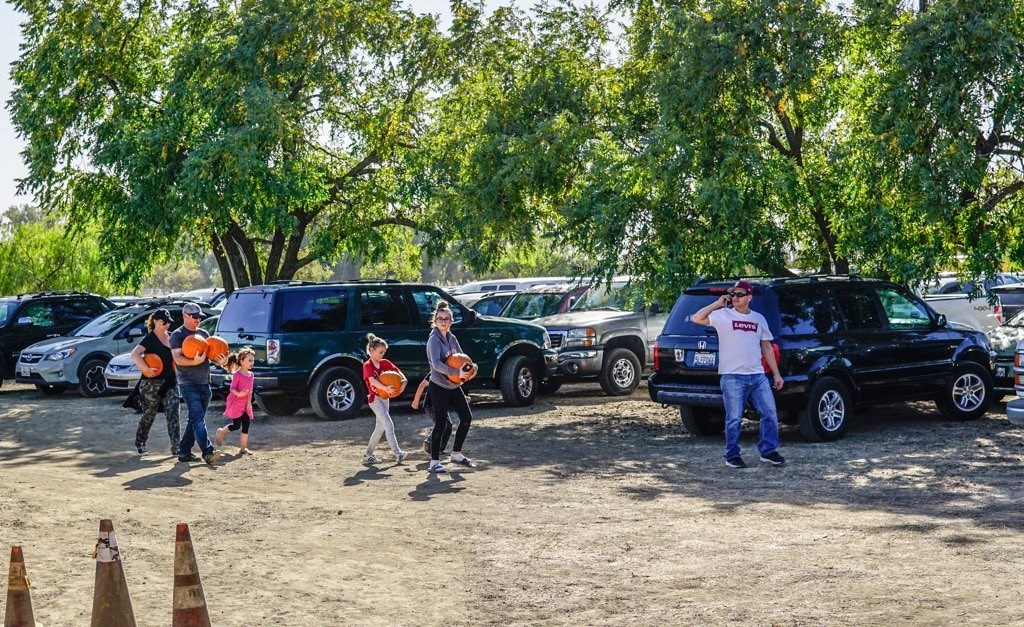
[206,336,229,362]
[182,334,208,359]
[445,353,474,383]
[142,354,163,377]
[376,370,404,398]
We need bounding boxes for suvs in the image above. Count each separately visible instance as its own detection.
[428,274,671,396]
[0,290,119,395]
[209,277,565,421]
[647,274,999,443]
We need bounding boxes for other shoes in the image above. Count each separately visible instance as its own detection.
[424,441,431,456]
[240,449,253,455]
[216,428,224,446]
[206,450,222,464]
[178,453,201,461]
[439,451,449,455]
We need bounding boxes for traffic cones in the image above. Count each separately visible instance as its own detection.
[90,518,137,627]
[3,545,35,627]
[172,523,211,627]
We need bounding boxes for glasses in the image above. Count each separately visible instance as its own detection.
[436,317,450,322]
[157,320,167,325]
[730,292,748,298]
[187,314,198,319]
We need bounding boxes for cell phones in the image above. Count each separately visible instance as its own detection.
[726,299,733,307]
[462,362,473,372]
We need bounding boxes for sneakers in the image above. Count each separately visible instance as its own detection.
[396,451,410,464]
[760,451,785,465]
[362,453,382,464]
[726,457,746,468]
[173,452,179,456]
[136,445,149,456]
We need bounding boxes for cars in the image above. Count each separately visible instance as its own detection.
[894,269,1024,429]
[14,287,239,398]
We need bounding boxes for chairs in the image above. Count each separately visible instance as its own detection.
[850,302,880,329]
[7,305,29,326]
[385,299,409,325]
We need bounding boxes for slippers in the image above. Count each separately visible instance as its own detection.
[451,457,476,468]
[428,464,446,473]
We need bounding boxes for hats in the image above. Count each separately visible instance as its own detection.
[182,303,207,317]
[727,280,753,296]
[153,309,174,323]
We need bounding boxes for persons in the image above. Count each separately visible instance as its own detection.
[412,300,477,473]
[169,303,230,466]
[691,280,786,468]
[132,308,182,456]
[363,333,409,466]
[216,347,259,455]
